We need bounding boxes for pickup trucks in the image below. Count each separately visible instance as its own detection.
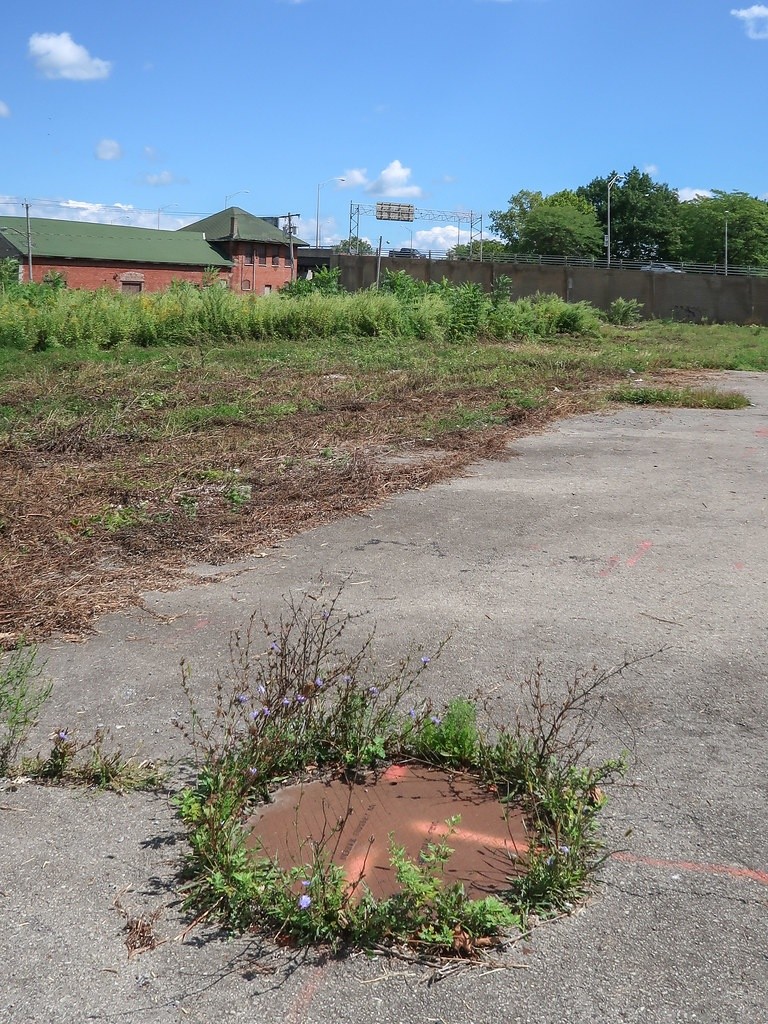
[388,248,426,259]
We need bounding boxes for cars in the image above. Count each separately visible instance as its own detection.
[641,262,686,274]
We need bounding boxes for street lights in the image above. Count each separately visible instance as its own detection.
[158,203,179,229]
[318,220,330,247]
[315,178,346,249]
[724,211,732,276]
[606,175,625,268]
[111,215,131,225]
[400,223,413,257]
[224,190,250,210]
